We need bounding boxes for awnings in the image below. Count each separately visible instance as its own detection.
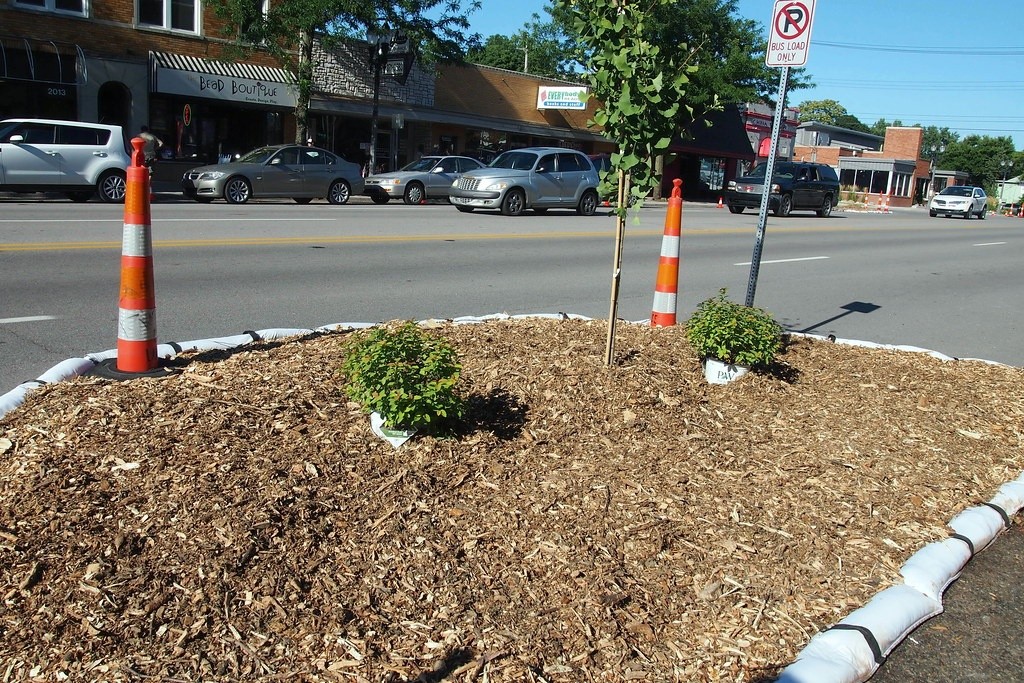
[148,51,310,109]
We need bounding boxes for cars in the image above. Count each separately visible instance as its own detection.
[449,147,602,216]
[362,154,487,205]
[180,144,366,205]
[586,153,612,174]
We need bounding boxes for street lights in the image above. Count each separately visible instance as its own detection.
[930,144,946,190]
[365,22,392,178]
[997,159,1014,215]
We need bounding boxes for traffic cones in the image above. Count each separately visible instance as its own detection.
[863,190,869,211]
[716,196,723,208]
[603,200,610,207]
[884,193,891,214]
[876,190,882,212]
[651,178,684,326]
[1019,204,1024,218]
[81,135,185,381]
[1007,202,1014,217]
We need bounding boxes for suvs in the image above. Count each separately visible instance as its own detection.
[725,160,839,218]
[930,186,988,219]
[0,118,132,203]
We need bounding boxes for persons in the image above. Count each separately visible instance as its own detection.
[415,144,424,160]
[306,138,314,146]
[137,126,163,195]
[429,144,456,156]
